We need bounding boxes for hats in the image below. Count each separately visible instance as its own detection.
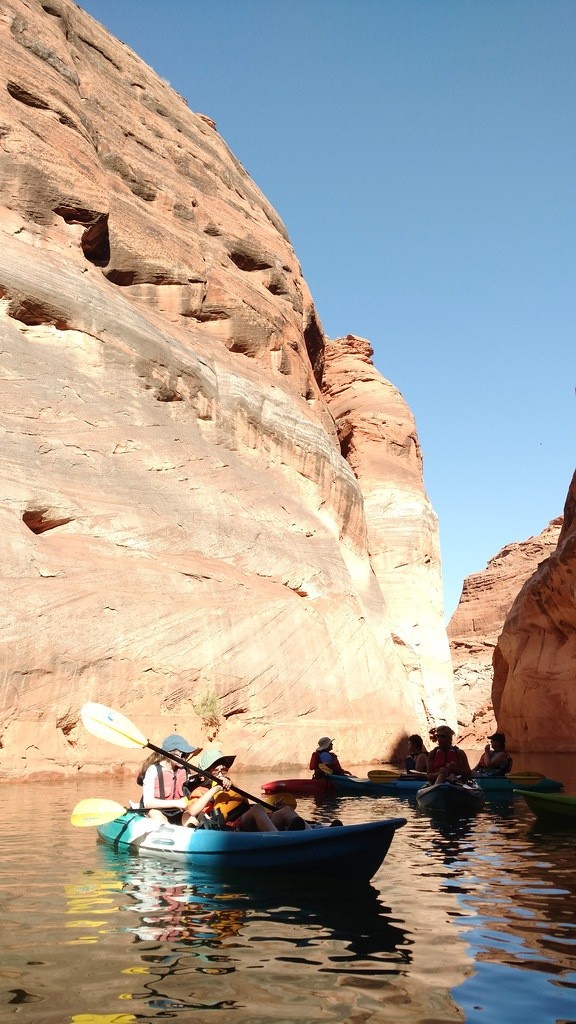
[487,733,506,742]
[316,737,336,750]
[189,750,237,780]
[161,734,197,753]
[435,726,455,736]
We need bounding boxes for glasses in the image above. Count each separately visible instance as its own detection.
[208,768,229,776]
[490,740,499,743]
[173,752,186,759]
[436,735,451,739]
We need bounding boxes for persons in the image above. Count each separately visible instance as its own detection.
[407,735,432,773]
[471,733,509,777]
[312,737,357,777]
[140,735,343,834]
[427,726,472,784]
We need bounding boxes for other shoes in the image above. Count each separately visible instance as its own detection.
[286,817,305,831]
[329,820,344,827]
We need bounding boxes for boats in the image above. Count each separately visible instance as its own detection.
[94,807,411,892]
[411,779,487,818]
[511,784,576,819]
[262,773,567,800]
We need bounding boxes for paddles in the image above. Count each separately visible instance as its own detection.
[69,792,298,828]
[367,769,547,788]
[80,701,281,813]
